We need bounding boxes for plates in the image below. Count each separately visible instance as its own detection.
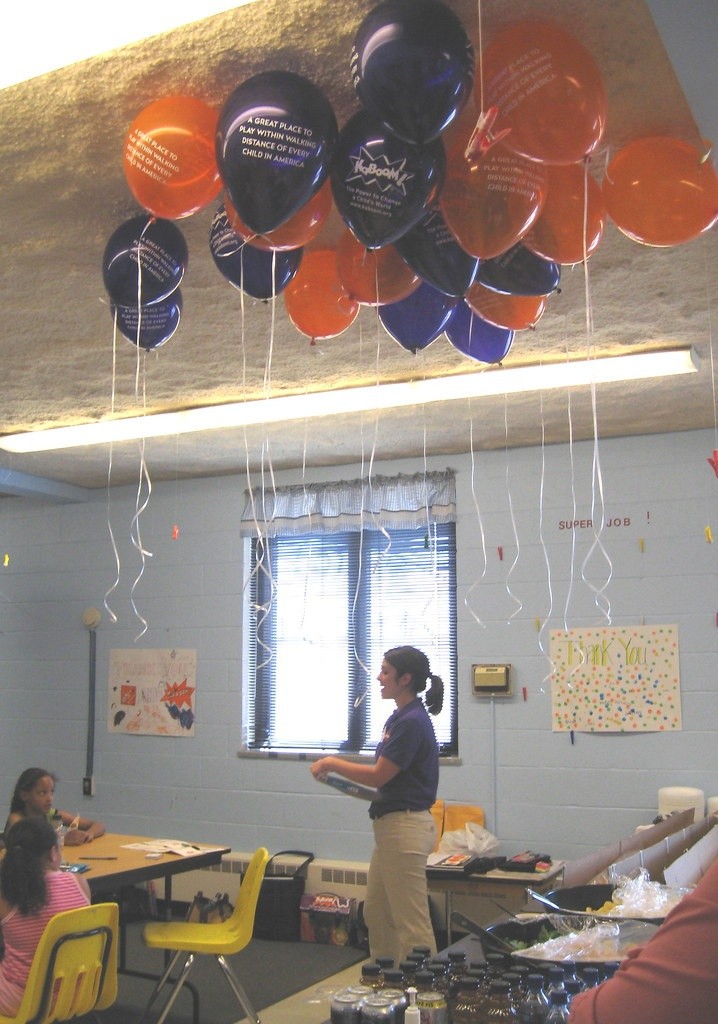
[658,786,718,825]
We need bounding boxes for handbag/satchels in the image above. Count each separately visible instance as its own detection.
[440,822,499,855]
[184,891,234,923]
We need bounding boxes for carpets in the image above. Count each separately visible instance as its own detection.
[52,913,368,1024]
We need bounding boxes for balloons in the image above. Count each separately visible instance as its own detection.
[442,241,563,363]
[376,282,457,354]
[209,204,302,303]
[350,0,478,147]
[474,24,607,164]
[336,230,422,305]
[603,137,718,248]
[398,205,478,297]
[103,215,188,308]
[437,132,550,263]
[509,162,607,265]
[285,248,360,344]
[224,179,334,253]
[333,112,448,253]
[123,96,223,219]
[217,70,338,237]
[111,286,182,352]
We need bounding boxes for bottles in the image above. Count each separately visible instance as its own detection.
[361,945,626,1024]
[49,814,64,860]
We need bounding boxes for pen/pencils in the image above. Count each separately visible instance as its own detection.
[78,856,118,860]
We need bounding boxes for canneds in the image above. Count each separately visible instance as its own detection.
[330,984,408,1024]
[416,991,448,1024]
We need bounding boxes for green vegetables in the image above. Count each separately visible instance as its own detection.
[501,924,561,950]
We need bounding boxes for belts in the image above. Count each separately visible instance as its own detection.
[371,809,418,820]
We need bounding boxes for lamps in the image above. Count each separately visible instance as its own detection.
[0,347,702,454]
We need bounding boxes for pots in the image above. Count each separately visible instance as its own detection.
[542,883,667,928]
[481,915,661,979]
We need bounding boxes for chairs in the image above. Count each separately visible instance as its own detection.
[137,846,268,1024]
[0,901,120,1024]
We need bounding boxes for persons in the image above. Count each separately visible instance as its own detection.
[310,646,444,967]
[0,818,91,1018]
[3,767,105,845]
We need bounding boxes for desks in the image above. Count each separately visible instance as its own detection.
[427,860,565,949]
[0,833,231,1024]
